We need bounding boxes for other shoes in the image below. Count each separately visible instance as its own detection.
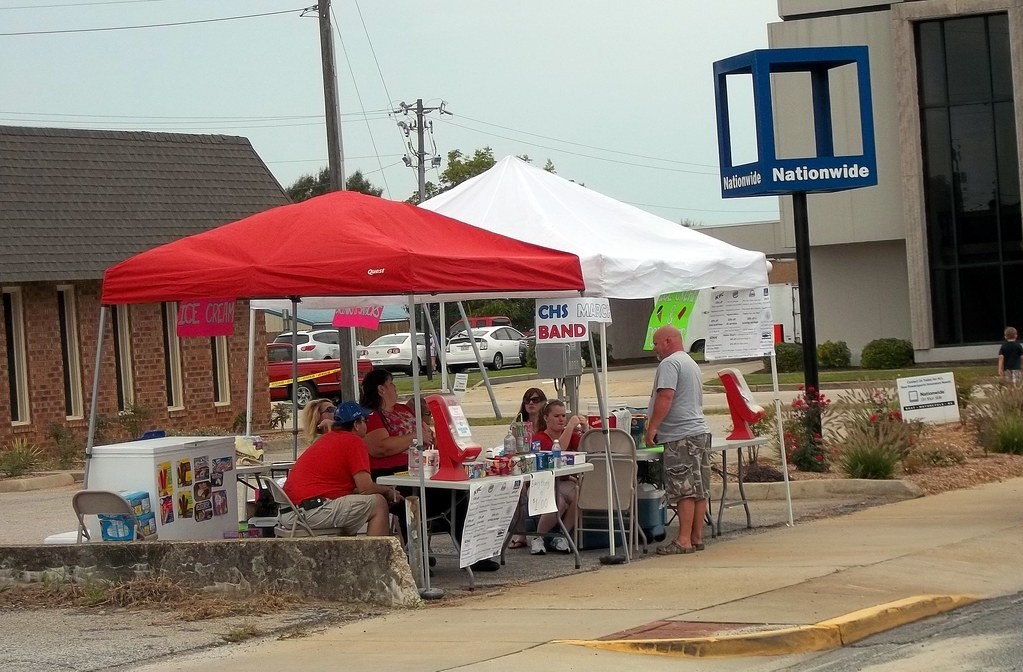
[462,559,500,572]
[691,543,705,550]
[423,566,433,577]
[656,540,696,554]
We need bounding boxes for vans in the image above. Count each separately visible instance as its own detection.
[449,317,513,339]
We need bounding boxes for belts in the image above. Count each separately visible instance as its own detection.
[279,505,303,514]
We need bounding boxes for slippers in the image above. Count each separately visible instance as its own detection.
[508,539,528,549]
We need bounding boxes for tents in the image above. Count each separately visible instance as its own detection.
[75,189,631,594]
[245,152,794,559]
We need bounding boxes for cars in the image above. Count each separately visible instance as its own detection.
[359,332,434,377]
[445,326,528,373]
[519,329,537,365]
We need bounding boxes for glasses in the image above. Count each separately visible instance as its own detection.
[359,417,367,423]
[524,397,541,404]
[544,398,563,411]
[425,411,431,416]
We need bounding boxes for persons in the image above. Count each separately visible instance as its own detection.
[531,398,590,554]
[277,400,404,536]
[998,327,1023,384]
[645,326,712,554]
[360,367,500,571]
[301,397,434,577]
[508,387,549,549]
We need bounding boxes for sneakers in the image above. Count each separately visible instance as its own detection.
[530,538,546,555]
[550,536,570,554]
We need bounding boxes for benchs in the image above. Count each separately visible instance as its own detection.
[268,347,293,362]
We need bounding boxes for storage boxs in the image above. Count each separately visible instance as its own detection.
[541,451,588,465]
[97,485,158,541]
[587,402,648,446]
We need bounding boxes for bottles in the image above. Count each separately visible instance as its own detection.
[552,439,561,469]
[504,430,516,454]
[408,439,420,477]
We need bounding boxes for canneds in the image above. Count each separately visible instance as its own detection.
[511,456,521,475]
[519,452,553,473]
[499,457,510,475]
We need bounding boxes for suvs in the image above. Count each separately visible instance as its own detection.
[272,329,367,360]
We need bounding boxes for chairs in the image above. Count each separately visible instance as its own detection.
[70,491,145,545]
[257,478,342,537]
[577,427,637,562]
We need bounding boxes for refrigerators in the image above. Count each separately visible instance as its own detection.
[86,434,239,542]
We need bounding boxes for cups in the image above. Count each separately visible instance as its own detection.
[423,444,439,479]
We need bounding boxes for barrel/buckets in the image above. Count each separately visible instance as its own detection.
[587,401,648,452]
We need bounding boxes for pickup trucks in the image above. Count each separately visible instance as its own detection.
[267,343,373,410]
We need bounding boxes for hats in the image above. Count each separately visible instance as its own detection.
[332,401,373,423]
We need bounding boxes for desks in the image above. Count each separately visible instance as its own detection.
[639,432,768,542]
[236,458,300,525]
[371,440,595,591]
